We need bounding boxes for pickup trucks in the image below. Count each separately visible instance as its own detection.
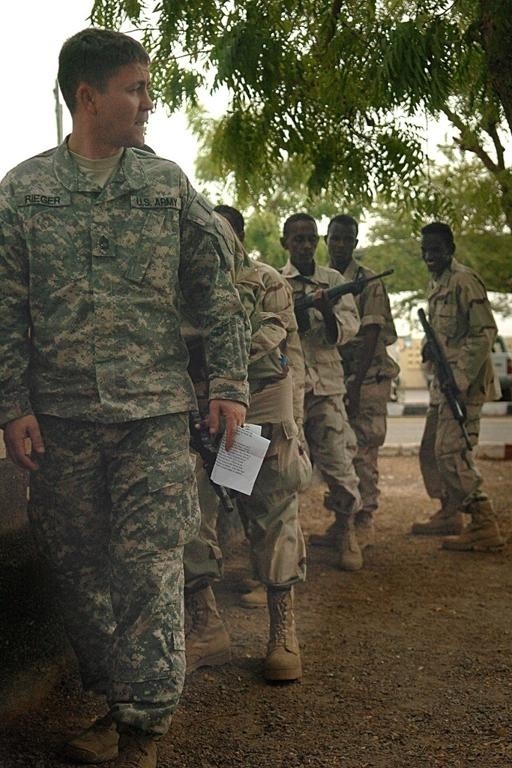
[489,334,511,401]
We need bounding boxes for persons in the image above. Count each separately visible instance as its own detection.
[3,27,251,767]
[277,214,400,571]
[184,206,312,681]
[408,224,505,555]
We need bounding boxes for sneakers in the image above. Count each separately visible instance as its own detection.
[239,578,268,608]
[116,730,158,768]
[63,715,119,763]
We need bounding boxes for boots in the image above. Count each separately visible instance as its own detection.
[443,500,506,551]
[183,586,233,674]
[263,588,301,680]
[413,498,463,534]
[310,510,374,571]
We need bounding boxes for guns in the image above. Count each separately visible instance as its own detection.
[294,266,394,332]
[418,308,473,451]
[185,335,237,513]
